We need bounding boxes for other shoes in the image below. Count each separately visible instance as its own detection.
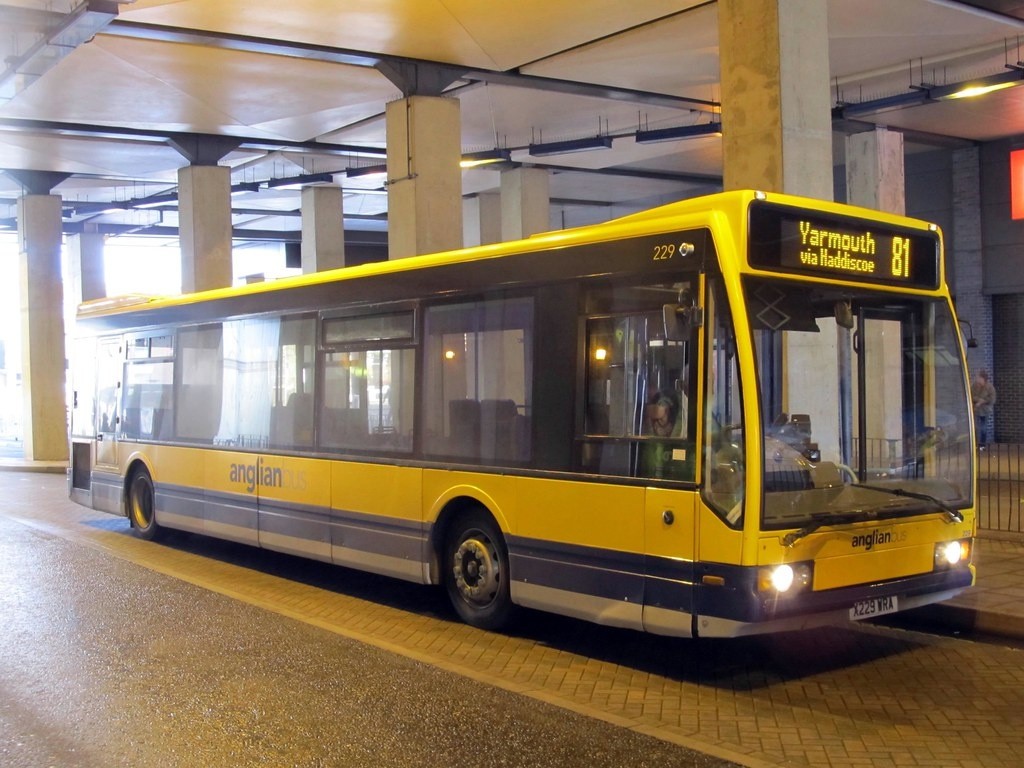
[979,447,985,451]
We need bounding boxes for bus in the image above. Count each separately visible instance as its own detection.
[66,187,980,640]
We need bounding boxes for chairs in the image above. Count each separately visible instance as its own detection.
[480,399,518,460]
[285,393,313,443]
[372,426,399,448]
[448,399,479,458]
[511,405,532,461]
[407,429,433,450]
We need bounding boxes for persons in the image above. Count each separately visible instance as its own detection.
[970,372,997,451]
[640,362,743,489]
